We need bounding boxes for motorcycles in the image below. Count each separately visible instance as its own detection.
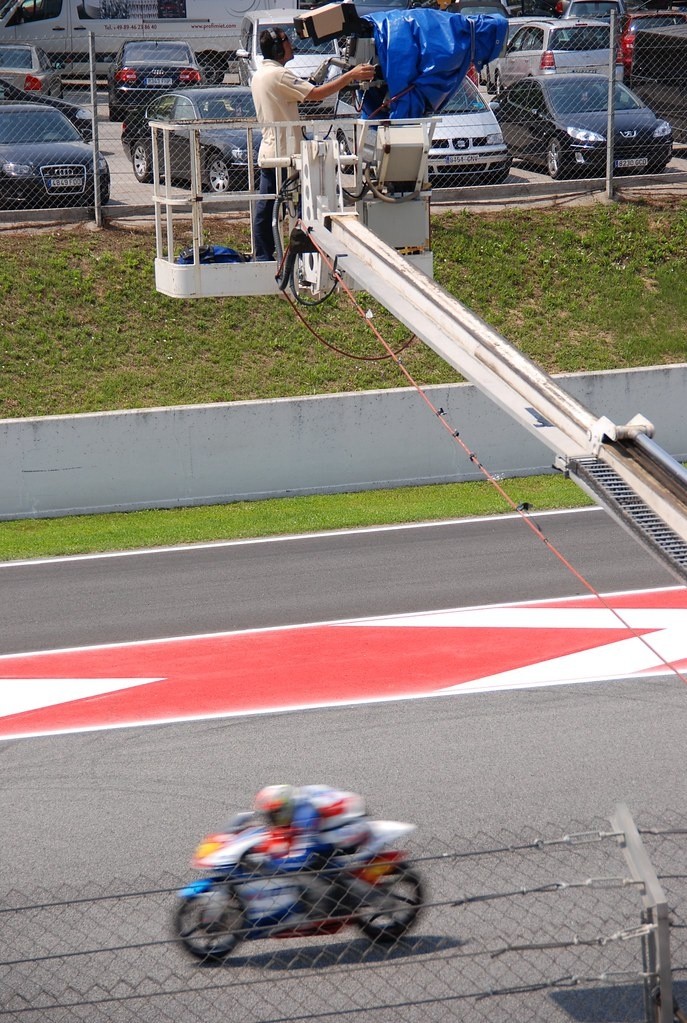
[170,783,426,959]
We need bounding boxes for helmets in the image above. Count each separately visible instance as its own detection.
[257,786,296,827]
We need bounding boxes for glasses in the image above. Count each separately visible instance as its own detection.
[280,35,289,42]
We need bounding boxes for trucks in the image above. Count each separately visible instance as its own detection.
[0,0,297,89]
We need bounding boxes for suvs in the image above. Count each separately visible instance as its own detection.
[104,39,206,122]
[0,42,67,100]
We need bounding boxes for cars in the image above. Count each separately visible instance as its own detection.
[334,0,687,95]
[0,77,94,142]
[120,88,331,192]
[333,74,508,191]
[0,102,111,211]
[489,74,674,182]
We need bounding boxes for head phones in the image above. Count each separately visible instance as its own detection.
[267,27,285,60]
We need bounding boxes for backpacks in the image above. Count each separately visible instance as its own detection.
[179,247,251,265]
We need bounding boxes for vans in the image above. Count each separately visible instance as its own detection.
[234,8,348,117]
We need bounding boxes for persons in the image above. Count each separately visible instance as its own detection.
[251,27,376,262]
[22,0,43,23]
[232,785,371,929]
